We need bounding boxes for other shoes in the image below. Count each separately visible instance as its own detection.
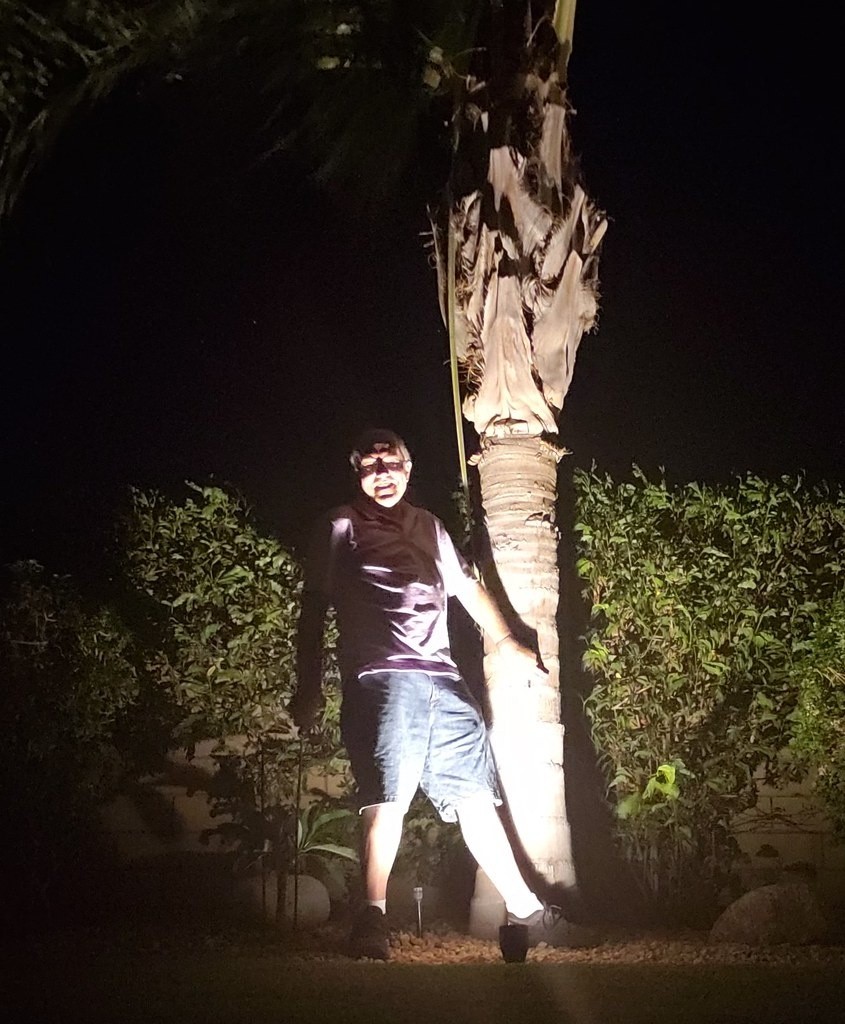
[507,900,602,948]
[352,906,391,962]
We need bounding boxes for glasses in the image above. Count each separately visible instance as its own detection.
[357,455,409,474]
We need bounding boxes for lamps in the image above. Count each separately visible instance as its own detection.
[500,924,529,966]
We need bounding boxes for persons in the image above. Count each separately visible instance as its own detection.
[290,431,605,958]
